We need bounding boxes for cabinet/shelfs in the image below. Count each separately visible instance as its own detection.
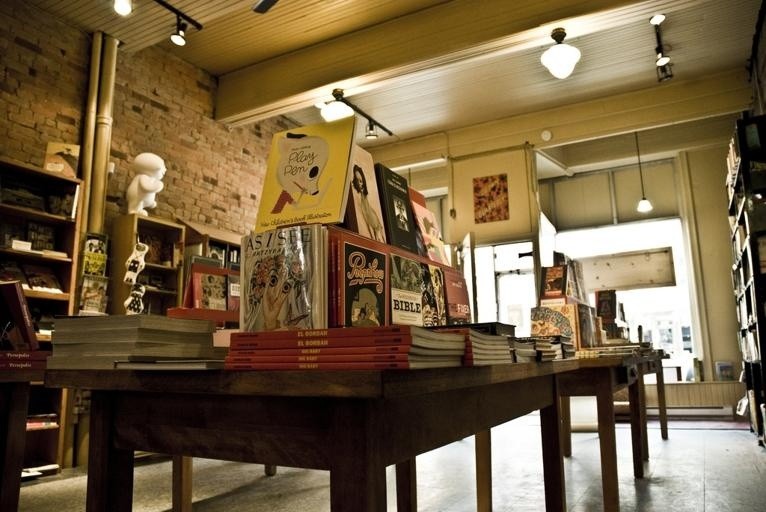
[79,210,241,328]
[1,159,86,478]
[724,113,766,451]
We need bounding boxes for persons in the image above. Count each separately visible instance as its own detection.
[348,162,386,244]
[392,199,410,233]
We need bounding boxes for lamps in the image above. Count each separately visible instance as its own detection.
[540,28,582,81]
[342,98,394,140]
[112,0,205,47]
[630,131,654,214]
[319,88,354,123]
[648,14,673,84]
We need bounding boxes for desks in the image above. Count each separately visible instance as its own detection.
[38,358,580,512]
[560,347,681,511]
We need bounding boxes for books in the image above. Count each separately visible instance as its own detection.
[341,140,389,244]
[253,112,359,236]
[0,180,111,482]
[375,161,424,257]
[532,252,653,359]
[410,200,451,270]
[47,215,556,374]
[404,186,428,257]
[724,119,766,448]
